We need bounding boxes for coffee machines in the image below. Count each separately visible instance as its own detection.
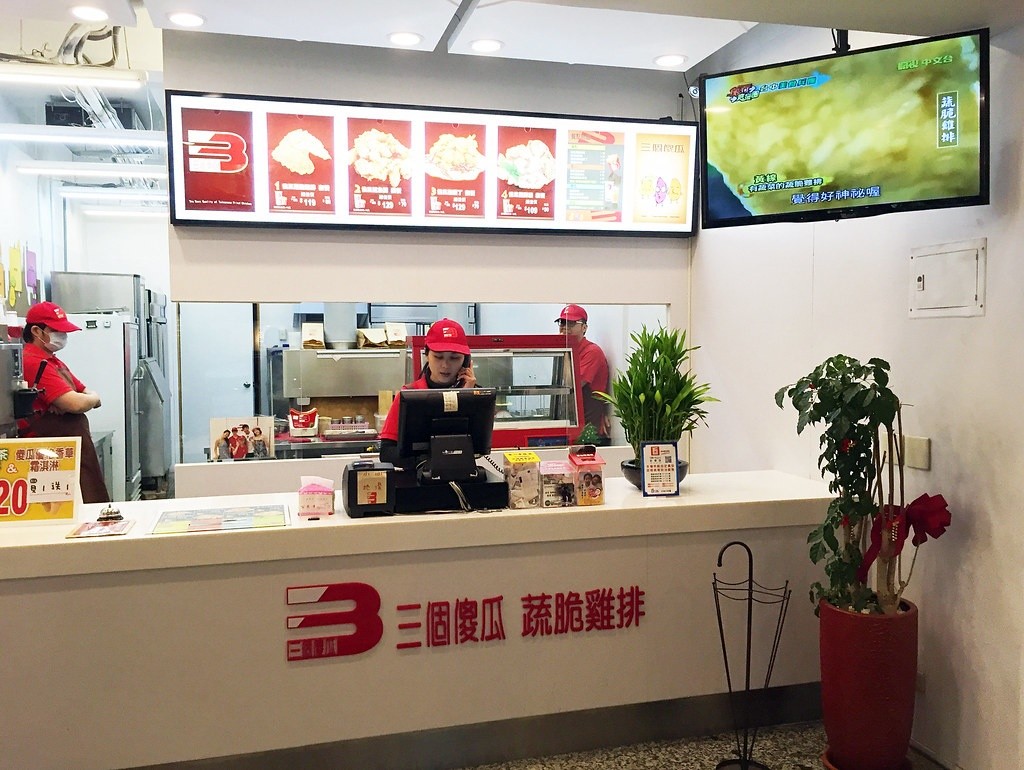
[0,344,46,438]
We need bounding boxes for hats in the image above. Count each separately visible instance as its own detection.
[424,317,471,355]
[25,302,83,333]
[554,305,588,321]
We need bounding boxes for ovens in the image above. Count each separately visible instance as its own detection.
[366,303,476,337]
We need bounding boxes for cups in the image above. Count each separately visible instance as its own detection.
[331,419,341,424]
[342,417,352,424]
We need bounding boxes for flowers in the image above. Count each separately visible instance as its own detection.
[871,491,952,556]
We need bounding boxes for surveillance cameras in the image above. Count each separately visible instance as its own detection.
[687,72,709,98]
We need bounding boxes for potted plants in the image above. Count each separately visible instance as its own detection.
[775,355,920,770]
[595,322,721,491]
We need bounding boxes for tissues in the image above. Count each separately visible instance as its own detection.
[297,475,336,516]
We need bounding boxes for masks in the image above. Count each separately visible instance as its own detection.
[32,327,68,352]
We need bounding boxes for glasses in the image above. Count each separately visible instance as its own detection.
[558,320,584,327]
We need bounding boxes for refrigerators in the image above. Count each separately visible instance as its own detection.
[54,313,143,502]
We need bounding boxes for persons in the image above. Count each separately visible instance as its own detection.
[214,430,231,459]
[583,473,602,490]
[228,427,248,458]
[380,318,477,468]
[13,301,110,502]
[251,428,267,457]
[554,305,610,445]
[241,424,254,457]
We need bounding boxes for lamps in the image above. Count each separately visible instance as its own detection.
[689,73,707,98]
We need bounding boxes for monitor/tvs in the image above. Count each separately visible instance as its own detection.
[397,388,497,483]
[700,28,991,228]
